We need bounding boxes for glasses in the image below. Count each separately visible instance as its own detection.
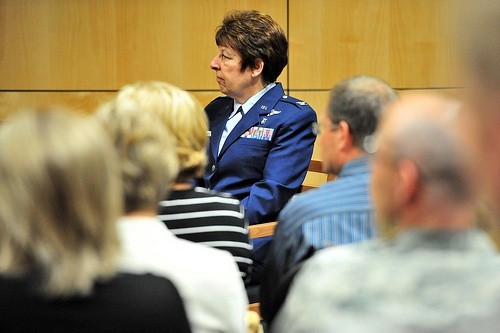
[311,120,353,135]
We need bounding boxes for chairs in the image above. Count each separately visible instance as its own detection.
[248,160,337,239]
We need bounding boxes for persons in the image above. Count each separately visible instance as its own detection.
[0,0,500,333]
[190,10,318,287]
